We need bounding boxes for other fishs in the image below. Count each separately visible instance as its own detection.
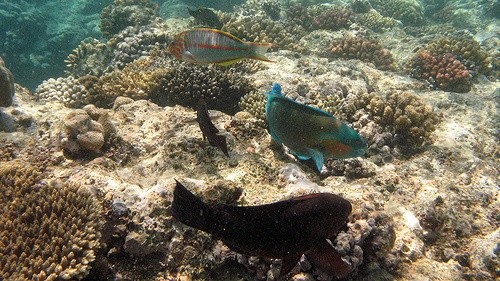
[168,178,353,281]
[263,82,368,172]
[197,98,230,157]
[187,6,223,30]
[167,27,281,68]
